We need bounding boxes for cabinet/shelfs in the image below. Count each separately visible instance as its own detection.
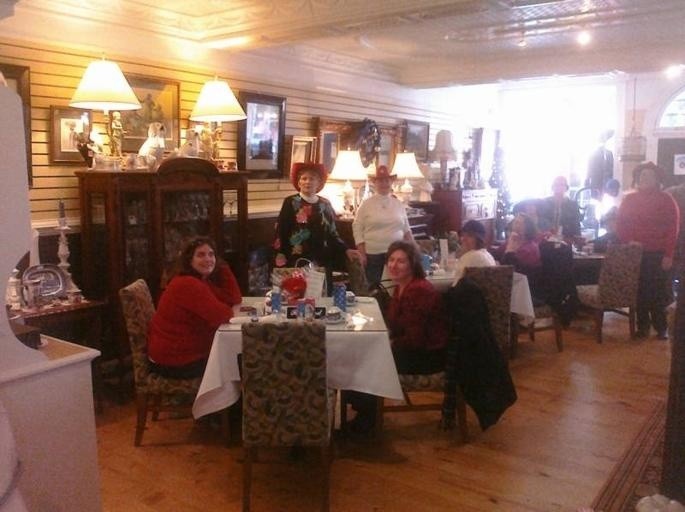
[432,188,498,243]
[334,211,434,248]
[75,158,251,401]
[0,76,104,511]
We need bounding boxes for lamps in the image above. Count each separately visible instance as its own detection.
[391,152,425,207]
[71,57,144,172]
[329,148,368,216]
[426,129,458,186]
[189,76,248,170]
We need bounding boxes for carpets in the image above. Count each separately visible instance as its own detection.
[590,396,668,511]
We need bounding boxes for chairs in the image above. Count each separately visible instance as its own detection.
[240,322,333,512]
[119,235,513,448]
[513,240,643,353]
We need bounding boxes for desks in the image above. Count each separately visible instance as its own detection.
[11,297,106,370]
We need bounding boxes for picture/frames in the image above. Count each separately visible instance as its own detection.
[402,118,431,164]
[234,89,289,180]
[46,104,94,166]
[312,114,379,179]
[290,134,318,166]
[108,73,183,152]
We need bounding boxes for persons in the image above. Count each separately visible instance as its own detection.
[110,111,128,158]
[585,143,614,203]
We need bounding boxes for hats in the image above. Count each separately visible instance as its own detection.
[367,165,397,181]
[629,161,664,186]
[290,160,327,193]
[552,175,569,190]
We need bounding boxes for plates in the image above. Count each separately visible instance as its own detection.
[21,263,64,299]
[239,287,357,325]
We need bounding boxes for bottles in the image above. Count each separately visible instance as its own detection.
[332,272,347,312]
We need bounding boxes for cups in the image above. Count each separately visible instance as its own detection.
[90,150,154,172]
[66,289,86,304]
